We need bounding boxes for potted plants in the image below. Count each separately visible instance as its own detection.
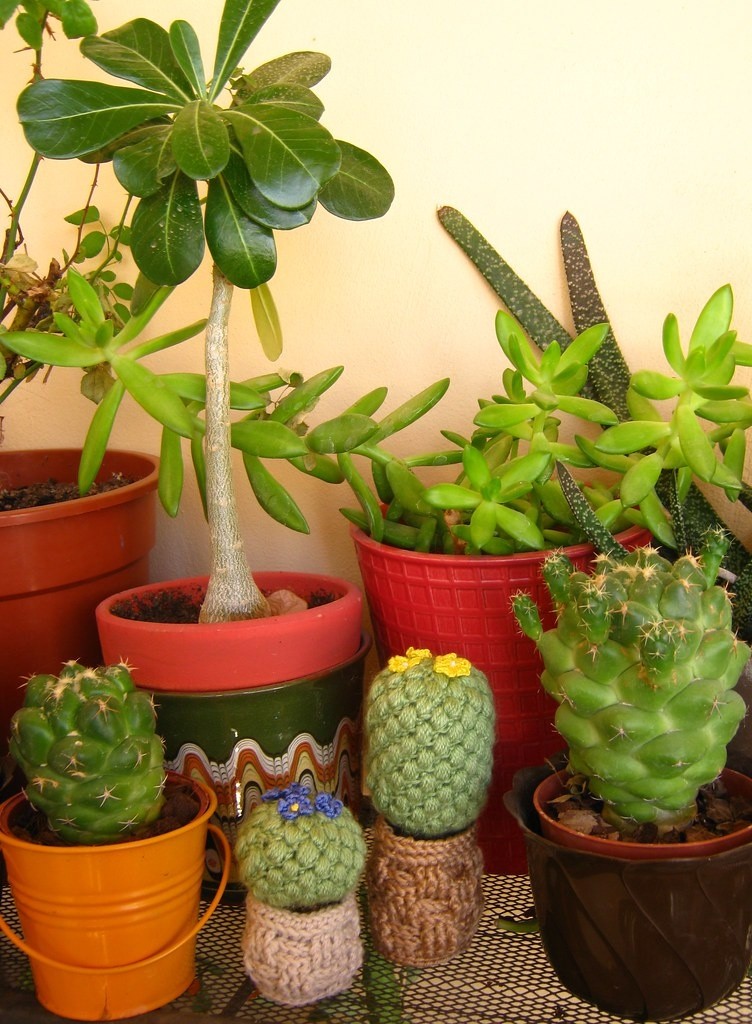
[0,0,752,1024]
